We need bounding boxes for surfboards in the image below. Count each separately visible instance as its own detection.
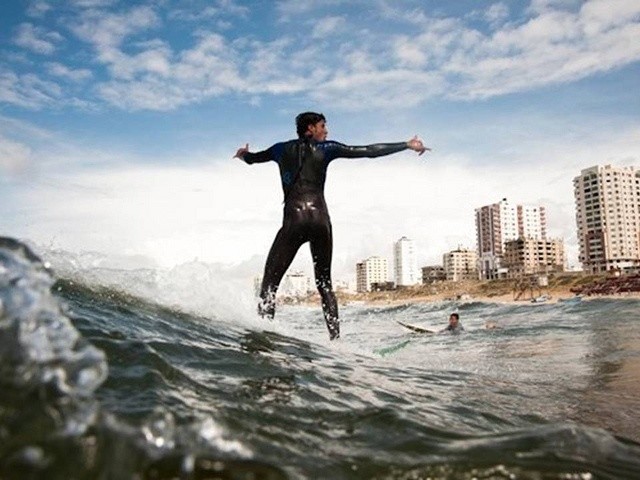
[396,321,434,333]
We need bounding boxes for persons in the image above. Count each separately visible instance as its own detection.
[439,313,464,333]
[232,111,432,342]
